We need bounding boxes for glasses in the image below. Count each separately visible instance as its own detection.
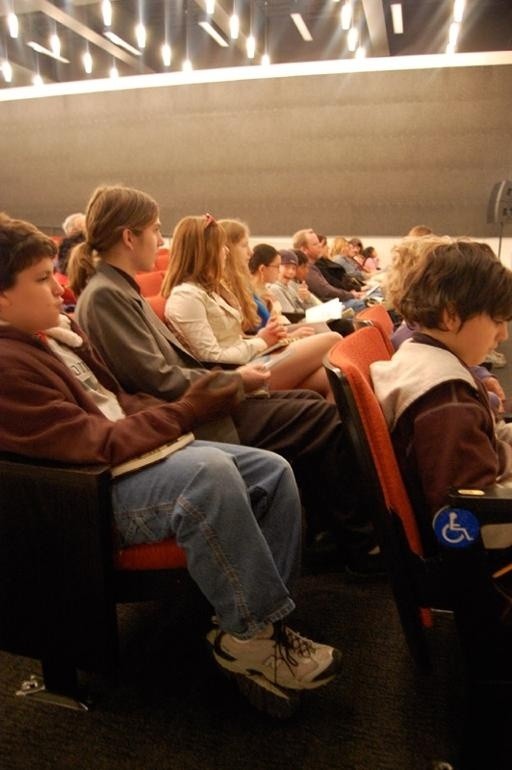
[205,213,218,230]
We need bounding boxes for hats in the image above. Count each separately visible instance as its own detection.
[279,249,297,266]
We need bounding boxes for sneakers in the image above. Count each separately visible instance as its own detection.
[213,625,348,691]
[207,628,298,706]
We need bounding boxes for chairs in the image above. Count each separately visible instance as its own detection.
[320,304,512,672]
[0,250,196,703]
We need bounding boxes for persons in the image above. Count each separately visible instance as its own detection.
[158,211,511,417]
[58,212,89,277]
[2,210,343,707]
[448,512,476,542]
[66,185,342,554]
[374,237,511,566]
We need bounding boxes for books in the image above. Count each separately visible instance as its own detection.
[110,431,195,479]
